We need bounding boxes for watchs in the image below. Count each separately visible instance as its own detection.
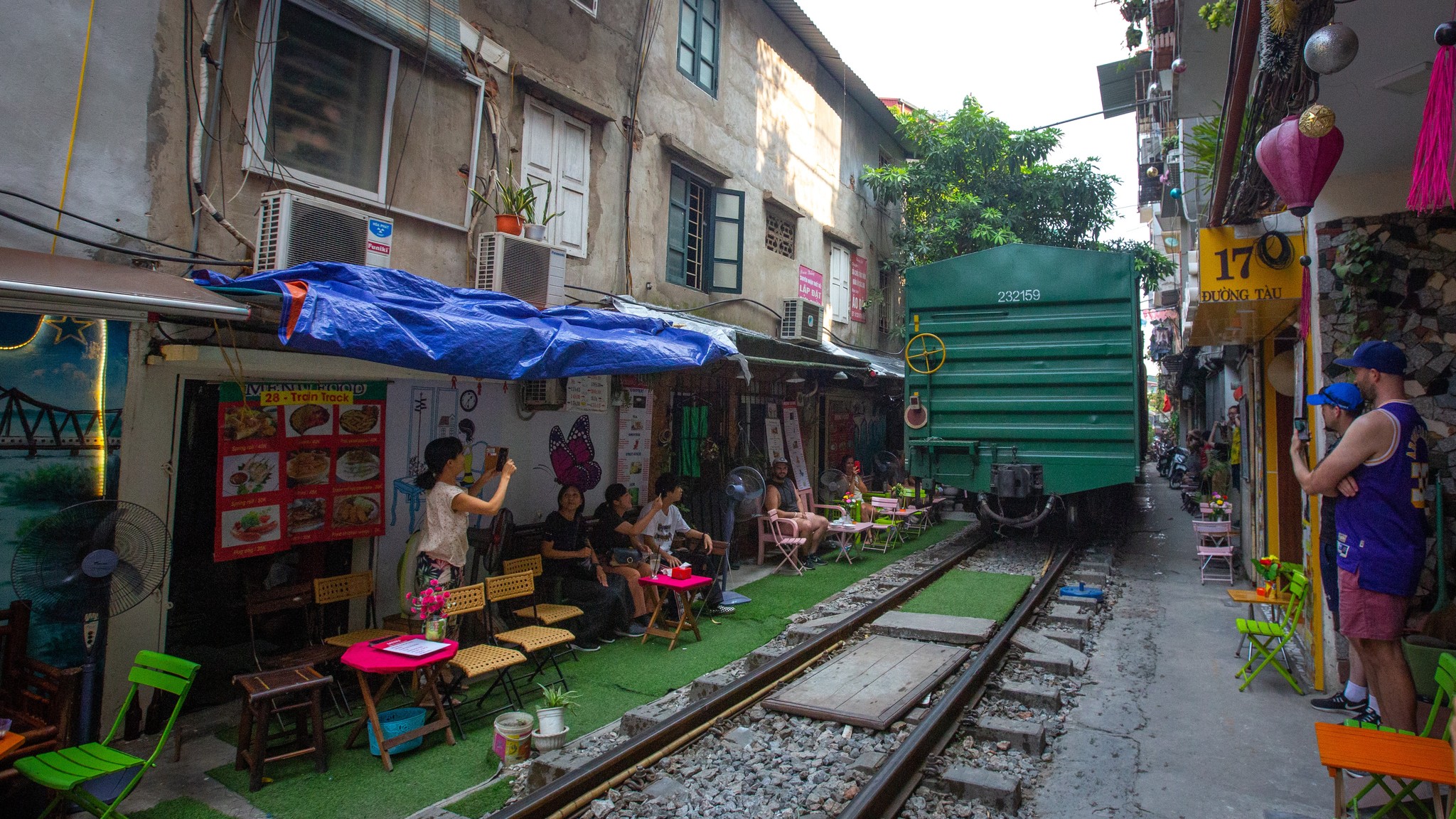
[856,482,859,487]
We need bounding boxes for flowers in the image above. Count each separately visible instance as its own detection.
[838,491,859,507]
[405,579,455,620]
[891,483,906,499]
[1209,492,1230,517]
[1253,553,1281,582]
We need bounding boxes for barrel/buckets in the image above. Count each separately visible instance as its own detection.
[493,710,535,767]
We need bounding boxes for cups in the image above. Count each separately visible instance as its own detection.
[650,553,660,579]
[844,521,852,524]
[841,516,847,521]
[833,520,840,524]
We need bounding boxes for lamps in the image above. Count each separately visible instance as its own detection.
[737,364,848,382]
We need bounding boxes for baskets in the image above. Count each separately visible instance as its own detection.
[366,706,426,755]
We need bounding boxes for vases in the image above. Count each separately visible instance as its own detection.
[424,615,447,643]
[846,507,852,521]
[896,498,900,512]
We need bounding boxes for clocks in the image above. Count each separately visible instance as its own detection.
[459,389,478,412]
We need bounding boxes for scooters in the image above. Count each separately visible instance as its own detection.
[1149,444,1192,489]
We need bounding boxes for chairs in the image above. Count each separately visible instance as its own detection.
[0,478,950,819]
[1192,500,1456,817]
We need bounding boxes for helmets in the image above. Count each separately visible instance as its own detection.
[772,457,788,465]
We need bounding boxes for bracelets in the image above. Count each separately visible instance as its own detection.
[702,533,705,541]
[794,511,797,518]
[593,563,601,570]
[851,480,855,482]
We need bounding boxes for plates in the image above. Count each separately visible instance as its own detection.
[227,471,249,486]
[334,497,379,526]
[287,513,324,534]
[337,452,380,483]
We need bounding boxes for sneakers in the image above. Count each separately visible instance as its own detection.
[1338,706,1379,728]
[810,552,828,565]
[1310,690,1368,716]
[800,555,815,569]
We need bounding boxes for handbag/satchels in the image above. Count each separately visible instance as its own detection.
[577,549,595,579]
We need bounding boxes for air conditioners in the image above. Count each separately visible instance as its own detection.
[252,188,393,273]
[524,379,566,405]
[473,232,566,310]
[781,297,824,345]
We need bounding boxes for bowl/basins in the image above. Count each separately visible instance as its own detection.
[286,456,330,485]
[843,524,855,529]
[883,507,893,510]
[829,523,842,528]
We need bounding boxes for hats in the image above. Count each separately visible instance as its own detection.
[1188,429,1202,438]
[1336,339,1407,376]
[1306,384,1362,409]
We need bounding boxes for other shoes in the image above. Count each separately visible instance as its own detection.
[592,623,615,643]
[434,673,469,690]
[673,619,692,631]
[1232,521,1240,529]
[872,532,879,541]
[705,603,736,616]
[637,613,664,629]
[419,691,461,706]
[1345,768,1370,778]
[615,617,646,637]
[566,635,601,652]
[865,537,872,545]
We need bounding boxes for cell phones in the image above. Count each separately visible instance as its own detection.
[496,447,509,471]
[854,461,860,473]
[659,493,662,498]
[1294,417,1310,442]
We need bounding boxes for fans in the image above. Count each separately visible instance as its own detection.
[870,451,900,496]
[814,468,849,550]
[465,508,513,650]
[12,499,173,808]
[697,467,765,607]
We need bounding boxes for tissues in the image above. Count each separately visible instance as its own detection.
[671,562,692,580]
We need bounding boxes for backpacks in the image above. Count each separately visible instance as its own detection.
[604,546,640,569]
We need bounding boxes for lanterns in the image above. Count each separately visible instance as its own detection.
[1256,116,1344,343]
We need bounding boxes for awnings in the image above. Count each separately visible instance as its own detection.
[0,246,920,386]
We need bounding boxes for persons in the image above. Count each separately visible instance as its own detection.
[1290,339,1429,777]
[542,484,646,652]
[1182,404,1241,530]
[1307,382,1383,727]
[1150,427,1177,462]
[592,485,662,628]
[636,473,735,630]
[838,454,880,546]
[872,448,943,530]
[414,437,517,705]
[763,457,828,569]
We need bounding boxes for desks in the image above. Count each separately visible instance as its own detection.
[1315,721,1456,819]
[879,508,919,544]
[827,522,874,565]
[638,571,712,652]
[920,496,946,528]
[342,636,460,772]
[1227,589,1301,672]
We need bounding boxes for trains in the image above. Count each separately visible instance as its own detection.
[902,242,1148,538]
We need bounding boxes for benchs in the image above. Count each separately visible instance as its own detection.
[497,506,691,631]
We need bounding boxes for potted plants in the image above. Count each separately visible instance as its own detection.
[463,160,565,242]
[535,681,581,736]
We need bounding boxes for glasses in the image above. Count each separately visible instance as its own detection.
[1318,386,1337,405]
